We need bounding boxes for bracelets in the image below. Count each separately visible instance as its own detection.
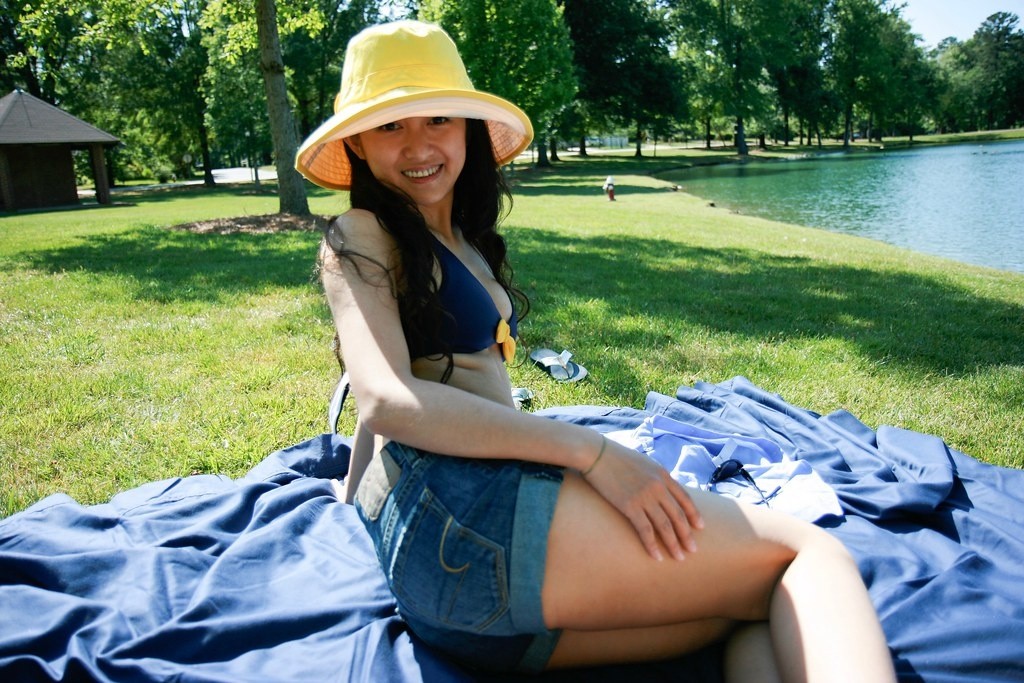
[581,435,606,475]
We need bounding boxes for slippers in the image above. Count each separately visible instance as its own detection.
[530,348,588,384]
[512,387,534,410]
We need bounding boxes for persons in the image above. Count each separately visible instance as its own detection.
[295,19,896,683]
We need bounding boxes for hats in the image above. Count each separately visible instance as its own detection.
[295,20,534,191]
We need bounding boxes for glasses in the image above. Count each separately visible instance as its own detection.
[706,459,781,509]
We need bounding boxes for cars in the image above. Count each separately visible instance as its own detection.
[240,156,261,168]
[585,136,603,148]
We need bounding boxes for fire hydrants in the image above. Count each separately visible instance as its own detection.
[603,175,615,200]
